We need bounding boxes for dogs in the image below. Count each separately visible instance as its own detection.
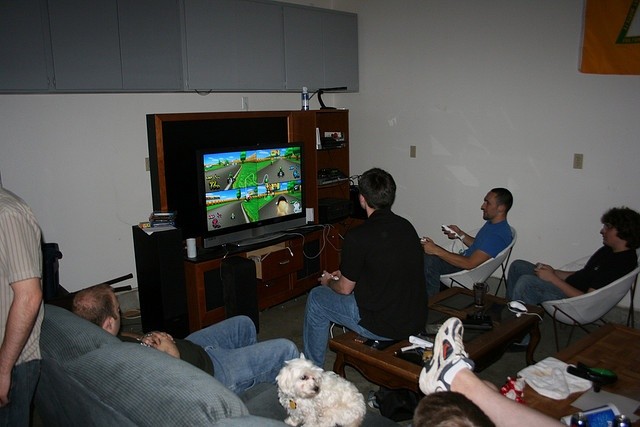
[274,352,367,426]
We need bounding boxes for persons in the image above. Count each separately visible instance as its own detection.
[507,206,639,307]
[0,181,44,427]
[418,317,567,426]
[302,167,429,368]
[71,284,301,395]
[422,188,513,299]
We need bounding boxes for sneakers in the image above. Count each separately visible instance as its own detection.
[418,317,475,397]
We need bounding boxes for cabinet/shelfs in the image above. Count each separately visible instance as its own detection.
[1,0,359,95]
[293,108,351,224]
[182,219,366,331]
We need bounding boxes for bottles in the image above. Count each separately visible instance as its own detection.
[301,87,310,110]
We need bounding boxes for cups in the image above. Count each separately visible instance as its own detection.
[473,282,490,308]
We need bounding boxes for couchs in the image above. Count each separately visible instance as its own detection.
[34,304,400,427]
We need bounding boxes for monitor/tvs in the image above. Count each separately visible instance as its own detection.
[195,142,306,248]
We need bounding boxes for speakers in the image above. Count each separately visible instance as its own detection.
[131,225,190,339]
[220,255,260,333]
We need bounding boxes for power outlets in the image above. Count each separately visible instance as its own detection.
[573,154,583,169]
[410,146,416,158]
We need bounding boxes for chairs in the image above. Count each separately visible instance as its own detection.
[541,249,639,352]
[440,225,517,297]
